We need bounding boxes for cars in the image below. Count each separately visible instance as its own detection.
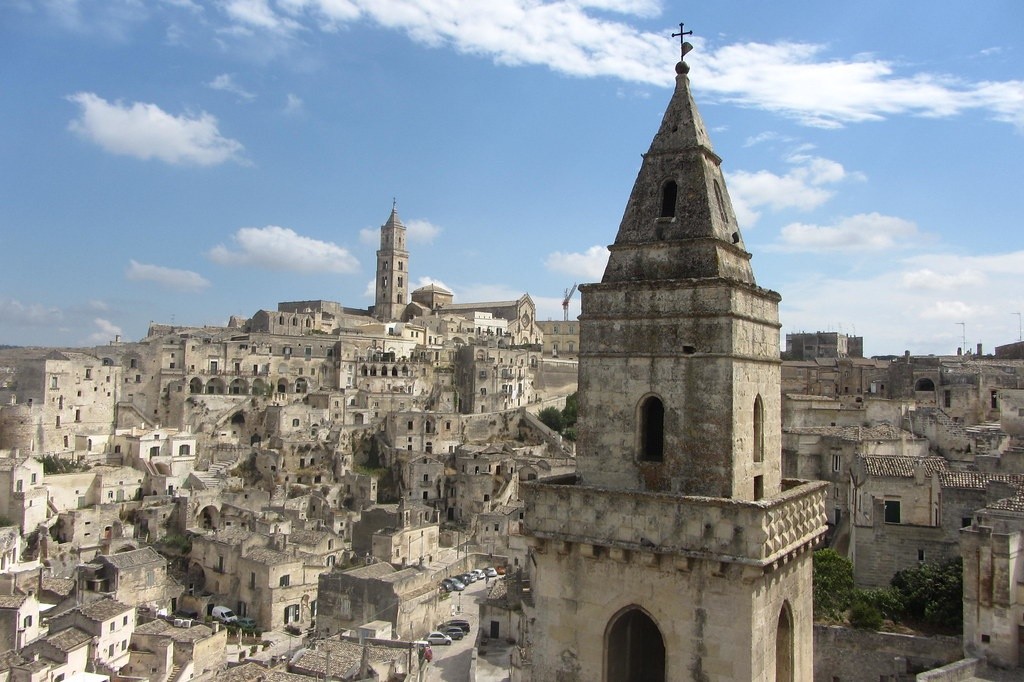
[483,566,498,577]
[420,644,433,663]
[442,577,465,591]
[461,573,478,583]
[453,574,470,586]
[471,569,486,580]
[440,625,463,640]
[496,566,505,575]
[235,618,257,629]
[427,632,453,646]
[440,581,454,593]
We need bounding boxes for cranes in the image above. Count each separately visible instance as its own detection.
[562,281,577,321]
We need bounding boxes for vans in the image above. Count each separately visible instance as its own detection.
[212,605,238,625]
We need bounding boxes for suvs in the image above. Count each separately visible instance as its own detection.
[437,619,470,636]
